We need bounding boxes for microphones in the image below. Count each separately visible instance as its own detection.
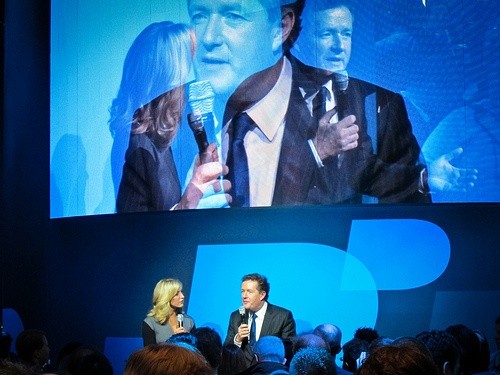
[189,80,221,150]
[333,70,350,122]
[178,313,184,329]
[239,307,248,346]
[185,109,221,180]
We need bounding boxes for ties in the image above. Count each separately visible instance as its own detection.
[312,86,339,181]
[223,112,256,207]
[250,313,257,343]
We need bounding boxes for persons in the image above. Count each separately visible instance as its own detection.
[50,0,500,220]
[0,274,500,375]
